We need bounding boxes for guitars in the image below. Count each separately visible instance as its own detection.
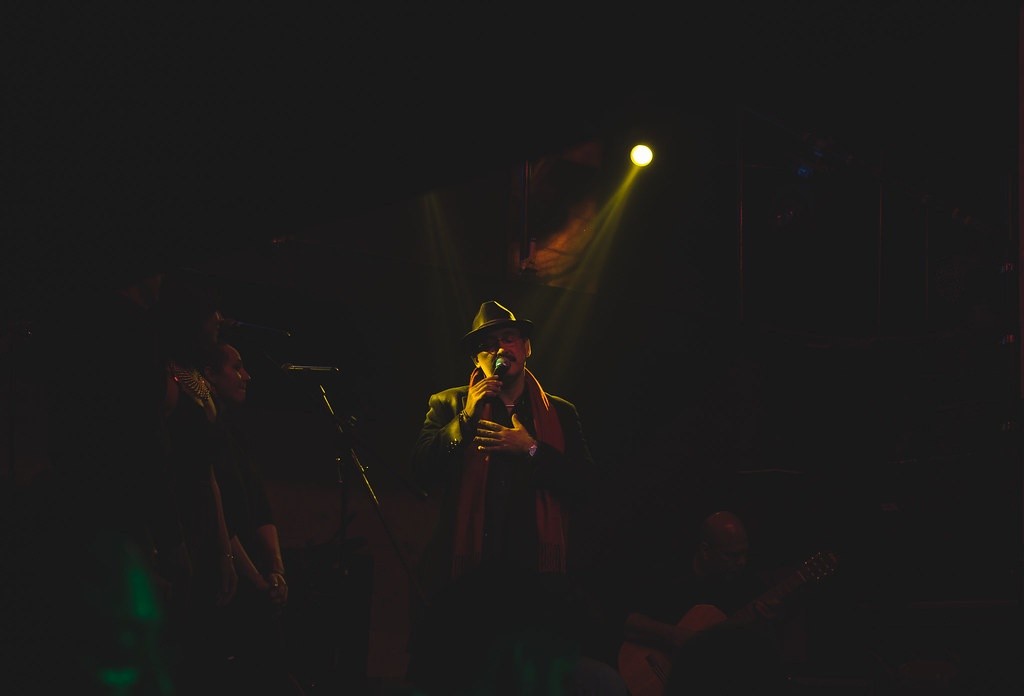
[614,549,843,696]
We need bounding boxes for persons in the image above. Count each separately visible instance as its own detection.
[122,268,292,696]
[623,510,795,696]
[407,299,603,696]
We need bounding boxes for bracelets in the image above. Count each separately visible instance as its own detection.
[462,409,473,427]
[222,552,234,560]
[271,568,285,576]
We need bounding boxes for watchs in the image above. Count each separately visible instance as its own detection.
[526,440,539,461]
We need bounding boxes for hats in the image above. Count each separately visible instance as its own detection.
[460,301,534,349]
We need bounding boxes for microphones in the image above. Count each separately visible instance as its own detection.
[222,319,294,337]
[492,357,511,380]
[280,363,340,377]
